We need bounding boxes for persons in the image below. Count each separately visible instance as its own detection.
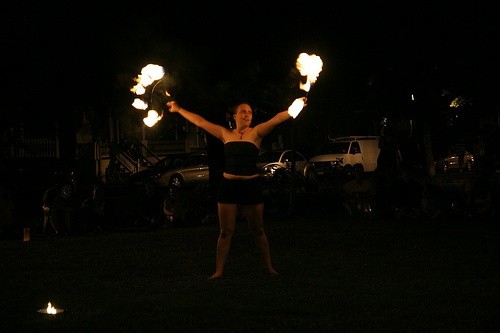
[337,163,440,223]
[166,96,308,280]
[37,182,219,237]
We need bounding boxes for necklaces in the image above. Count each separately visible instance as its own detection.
[236,129,247,139]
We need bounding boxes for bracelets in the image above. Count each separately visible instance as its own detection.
[177,106,182,112]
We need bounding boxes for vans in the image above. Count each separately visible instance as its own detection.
[308,136,382,175]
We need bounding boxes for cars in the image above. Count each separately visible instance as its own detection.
[150,152,210,188]
[258,149,308,176]
[433,150,478,175]
